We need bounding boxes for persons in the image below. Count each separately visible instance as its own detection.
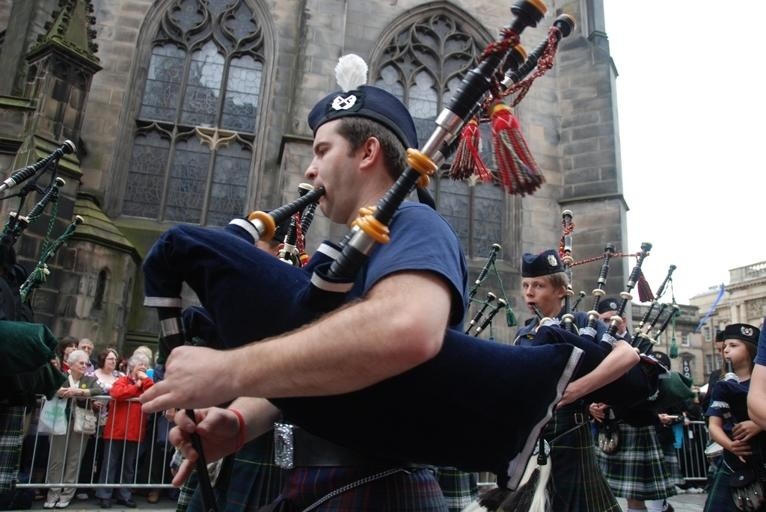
[136,83,473,511]
[509,249,641,512]
[1,297,765,511]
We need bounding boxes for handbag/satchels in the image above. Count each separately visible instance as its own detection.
[72,406,96,435]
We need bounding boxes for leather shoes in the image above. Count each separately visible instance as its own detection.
[146,489,160,503]
[101,497,112,508]
[118,498,137,509]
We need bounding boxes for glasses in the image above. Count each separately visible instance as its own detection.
[80,361,92,368]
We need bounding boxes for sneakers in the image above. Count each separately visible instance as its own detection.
[675,485,708,493]
[33,492,88,509]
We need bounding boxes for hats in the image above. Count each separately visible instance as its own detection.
[599,298,617,314]
[715,324,760,344]
[522,250,564,276]
[307,84,418,151]
[632,337,670,370]
